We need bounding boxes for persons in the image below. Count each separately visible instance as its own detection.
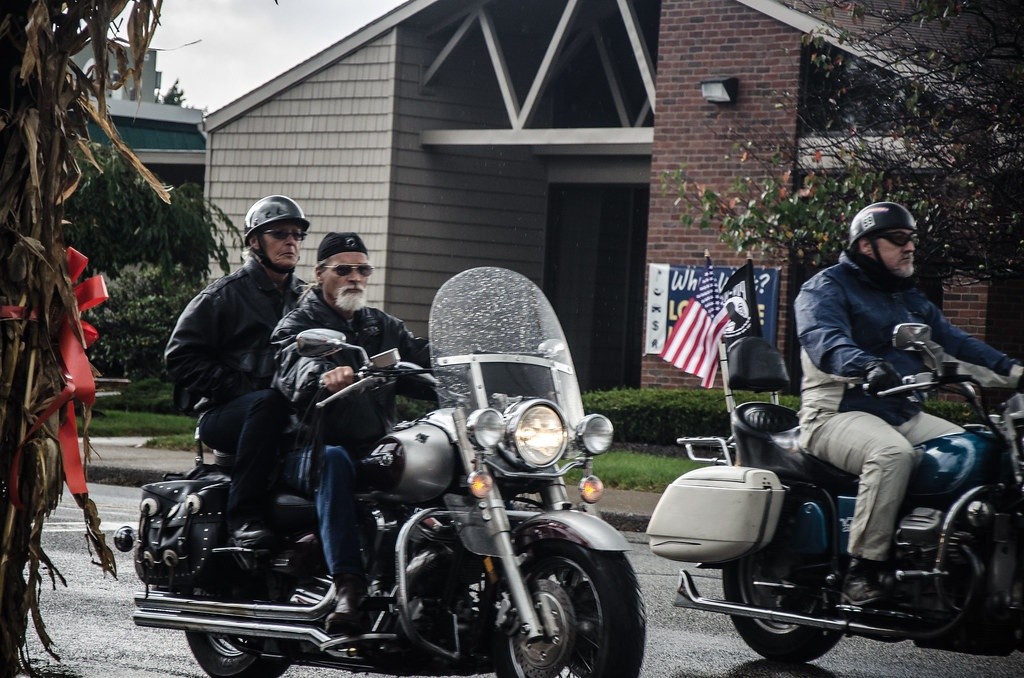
[163,195,473,635]
[794,202,1024,607]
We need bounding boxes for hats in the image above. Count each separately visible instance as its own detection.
[317,232,367,263]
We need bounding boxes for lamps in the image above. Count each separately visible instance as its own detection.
[700,77,738,104]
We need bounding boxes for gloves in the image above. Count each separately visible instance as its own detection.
[863,360,904,400]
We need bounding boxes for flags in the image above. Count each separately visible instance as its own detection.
[658,257,764,389]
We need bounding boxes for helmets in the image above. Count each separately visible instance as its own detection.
[847,201,917,252]
[244,195,310,248]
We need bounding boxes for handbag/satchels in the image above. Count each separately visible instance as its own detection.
[134,466,231,596]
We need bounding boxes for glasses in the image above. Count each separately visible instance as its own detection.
[256,230,307,241]
[322,264,375,276]
[872,230,921,247]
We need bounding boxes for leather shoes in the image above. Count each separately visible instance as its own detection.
[324,572,370,634]
[226,520,274,547]
[841,556,893,607]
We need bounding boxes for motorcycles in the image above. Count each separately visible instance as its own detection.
[114,267,646,678]
[645,322,1023,663]
